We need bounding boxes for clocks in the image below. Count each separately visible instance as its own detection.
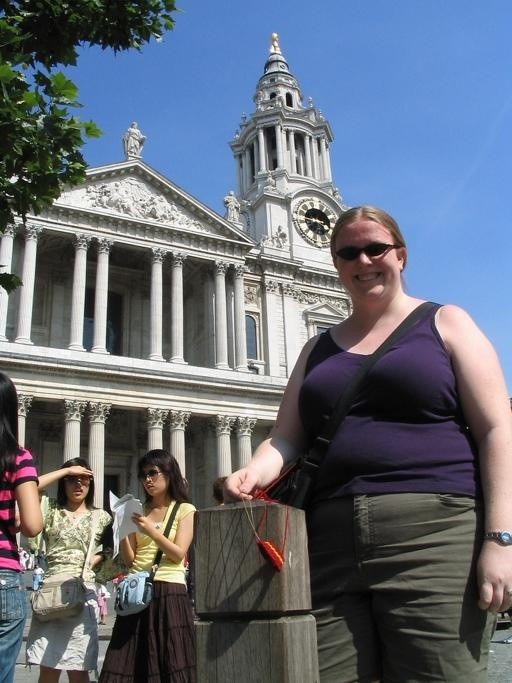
[293,196,337,248]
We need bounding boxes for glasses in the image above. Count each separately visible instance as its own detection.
[137,469,159,481]
[334,242,402,260]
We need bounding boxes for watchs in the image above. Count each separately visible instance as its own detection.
[482,531,512,546]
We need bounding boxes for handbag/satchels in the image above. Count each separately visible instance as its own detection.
[30,573,84,622]
[268,452,314,508]
[115,570,153,616]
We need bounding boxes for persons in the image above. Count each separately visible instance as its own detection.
[19,547,46,592]
[14,456,128,683]
[212,476,228,504]
[124,122,146,155]
[94,579,110,625]
[223,191,241,222]
[97,449,199,682]
[275,225,287,247]
[228,205,511,683]
[0,373,44,683]
